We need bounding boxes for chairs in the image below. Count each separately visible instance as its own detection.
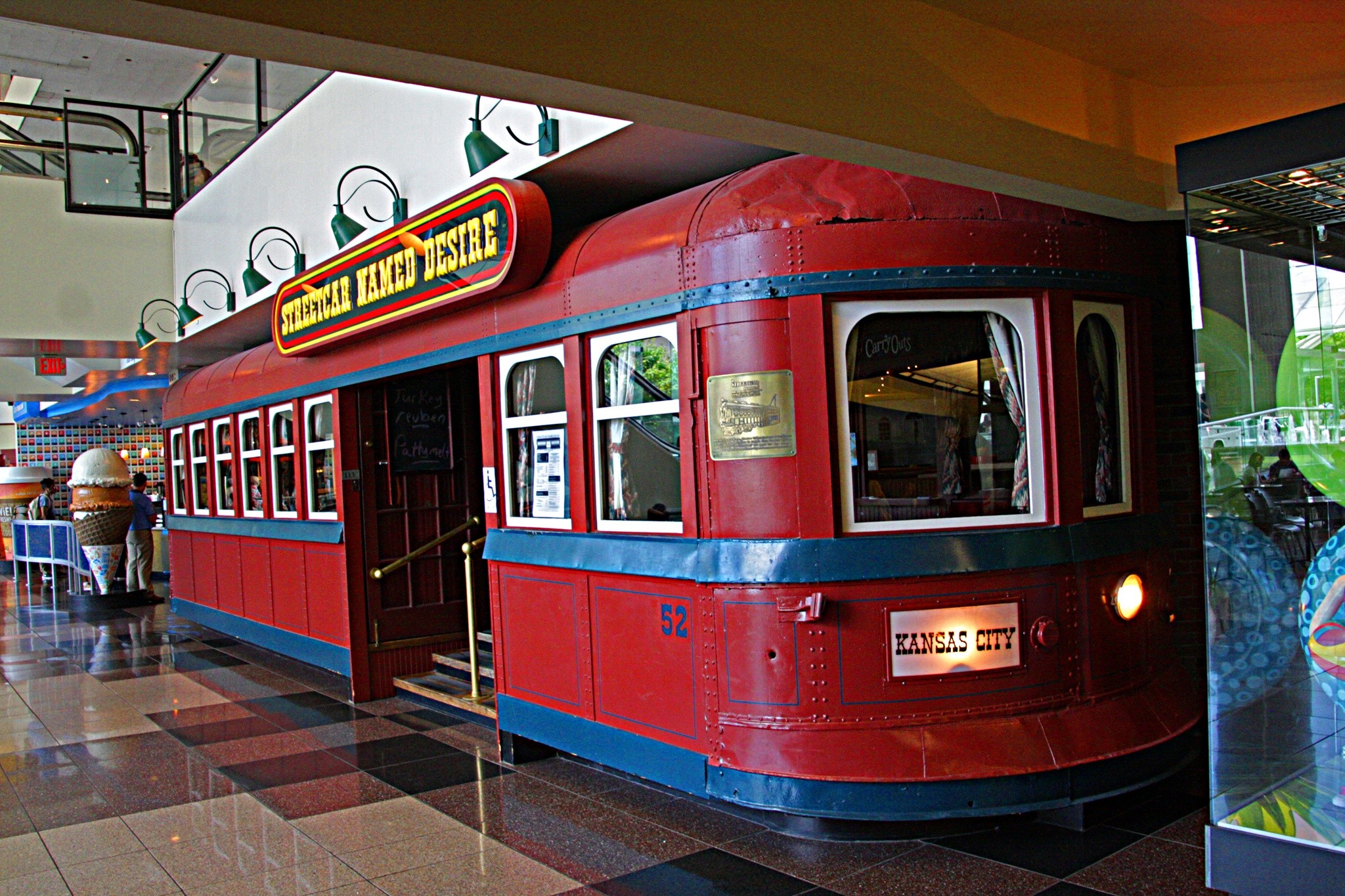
[1243,492,1304,565]
[1254,488,1317,562]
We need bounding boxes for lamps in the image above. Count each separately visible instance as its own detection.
[464,93,559,177]
[243,226,305,298]
[99,416,108,427]
[135,298,185,351]
[177,268,237,327]
[332,164,408,250]
[204,63,218,84]
[140,424,149,459]
[136,410,147,427]
[118,412,127,429]
[120,429,129,460]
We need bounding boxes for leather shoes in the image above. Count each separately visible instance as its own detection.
[143,595,165,602]
[126,591,144,599]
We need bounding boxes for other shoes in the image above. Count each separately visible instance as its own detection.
[42,573,52,580]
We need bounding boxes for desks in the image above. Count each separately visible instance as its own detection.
[1274,497,1342,556]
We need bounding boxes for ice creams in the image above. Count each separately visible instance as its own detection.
[65,445,132,594]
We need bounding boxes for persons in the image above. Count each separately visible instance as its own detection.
[128,606,160,709]
[1198,394,1345,516]
[36,478,68,580]
[227,479,233,510]
[125,473,165,602]
[250,476,262,510]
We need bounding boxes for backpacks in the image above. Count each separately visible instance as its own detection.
[27,494,51,520]
